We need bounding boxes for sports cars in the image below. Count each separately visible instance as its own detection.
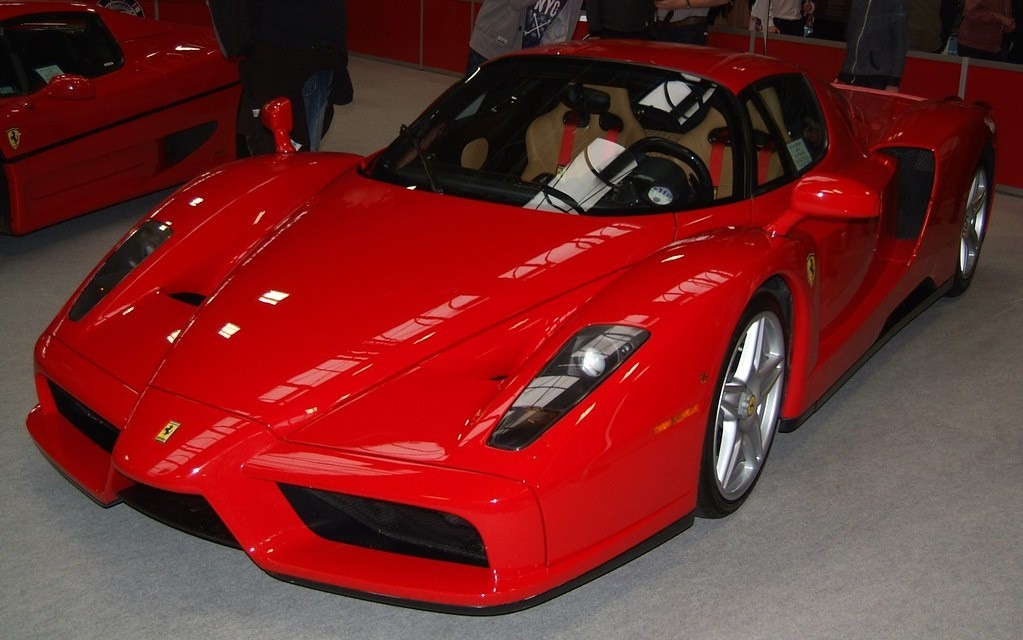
[23,35,1002,612]
[1,1,247,243]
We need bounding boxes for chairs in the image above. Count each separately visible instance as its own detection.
[668,84,794,204]
[523,79,648,190]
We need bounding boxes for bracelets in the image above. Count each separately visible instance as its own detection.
[686,1,692,10]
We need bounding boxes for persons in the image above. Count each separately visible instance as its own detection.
[204,0,355,162]
[751,0,816,38]
[829,0,909,94]
[956,0,1016,62]
[588,0,736,45]
[466,1,587,79]
